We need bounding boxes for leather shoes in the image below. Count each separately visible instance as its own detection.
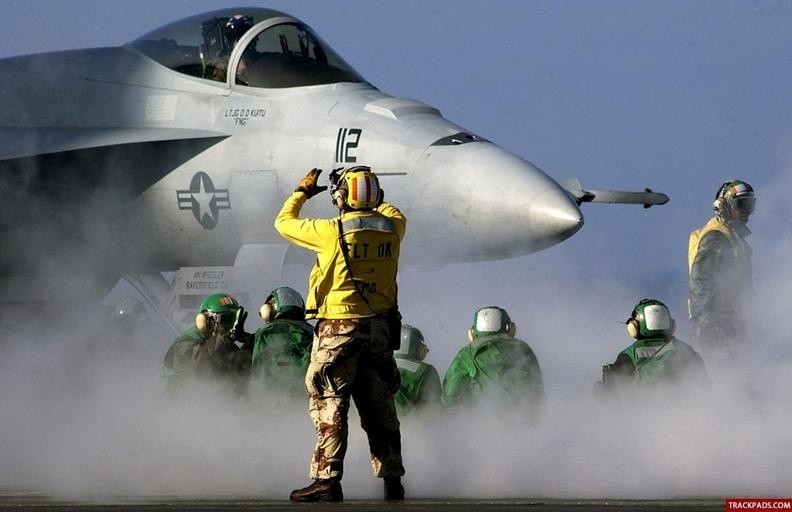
[290,478,345,504]
[384,480,406,502]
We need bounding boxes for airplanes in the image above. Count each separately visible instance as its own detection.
[0,6,668,342]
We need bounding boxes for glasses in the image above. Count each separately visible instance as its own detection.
[729,198,755,213]
[211,309,235,327]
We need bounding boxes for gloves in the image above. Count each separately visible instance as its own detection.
[229,306,249,342]
[295,168,328,200]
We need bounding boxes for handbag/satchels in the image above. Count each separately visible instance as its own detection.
[369,311,406,351]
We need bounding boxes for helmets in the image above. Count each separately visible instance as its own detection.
[471,306,510,339]
[337,165,382,212]
[718,180,755,217]
[635,300,673,339]
[393,321,425,361]
[200,292,244,336]
[270,287,306,321]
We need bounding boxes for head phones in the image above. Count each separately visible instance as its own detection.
[334,166,384,210]
[467,323,515,341]
[415,342,429,362]
[196,311,208,331]
[627,298,678,338]
[259,304,274,322]
[713,179,737,218]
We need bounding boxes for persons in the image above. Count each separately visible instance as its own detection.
[274,166,407,502]
[393,324,441,416]
[251,287,315,398]
[205,14,265,83]
[163,294,254,385]
[602,299,712,401]
[441,306,544,411]
[688,178,757,359]
